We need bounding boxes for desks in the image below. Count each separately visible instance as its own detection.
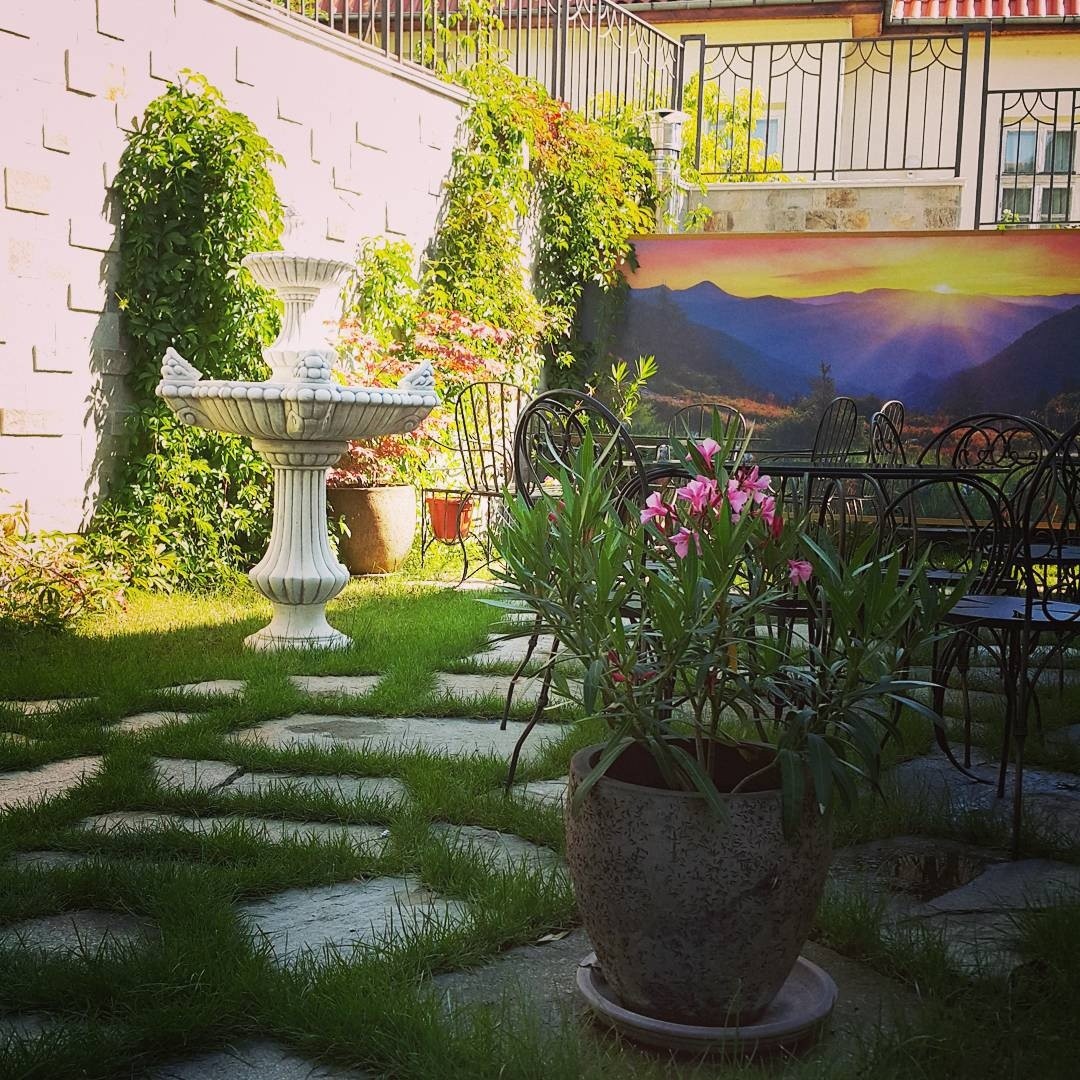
[1025,450,1080,525]
[614,445,868,461]
[559,424,772,508]
[658,463,1013,489]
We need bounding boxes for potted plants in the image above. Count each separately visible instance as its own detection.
[326,232,432,573]
[421,311,507,539]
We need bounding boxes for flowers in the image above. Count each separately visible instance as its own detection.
[475,409,986,828]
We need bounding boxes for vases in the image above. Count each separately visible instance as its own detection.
[568,736,844,1049]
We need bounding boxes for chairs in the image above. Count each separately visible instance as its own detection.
[455,382,1080,855]
